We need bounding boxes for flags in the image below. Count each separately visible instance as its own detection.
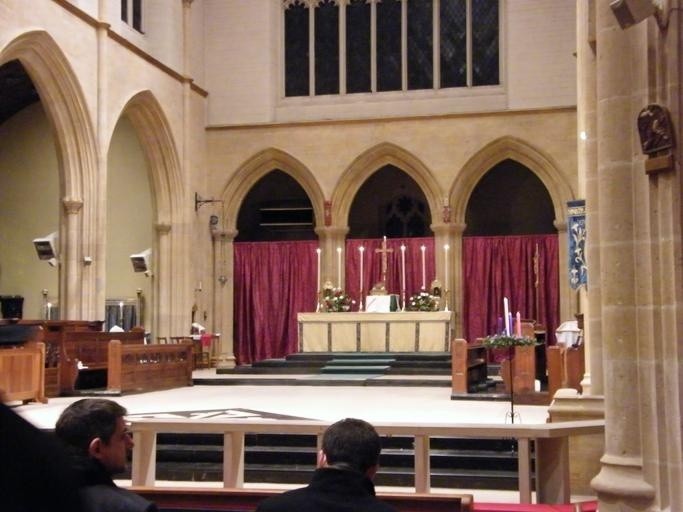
[565,198,588,294]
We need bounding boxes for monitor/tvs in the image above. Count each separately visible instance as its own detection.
[32,238,56,262]
[130,254,148,273]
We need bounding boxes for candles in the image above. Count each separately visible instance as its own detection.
[502,296,523,339]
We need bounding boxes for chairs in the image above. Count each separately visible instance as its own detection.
[157,336,210,370]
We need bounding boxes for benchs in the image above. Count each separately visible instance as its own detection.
[17,317,148,395]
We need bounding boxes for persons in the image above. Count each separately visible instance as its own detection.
[54,399,157,511]
[0,392,94,512]
[257,416,398,511]
